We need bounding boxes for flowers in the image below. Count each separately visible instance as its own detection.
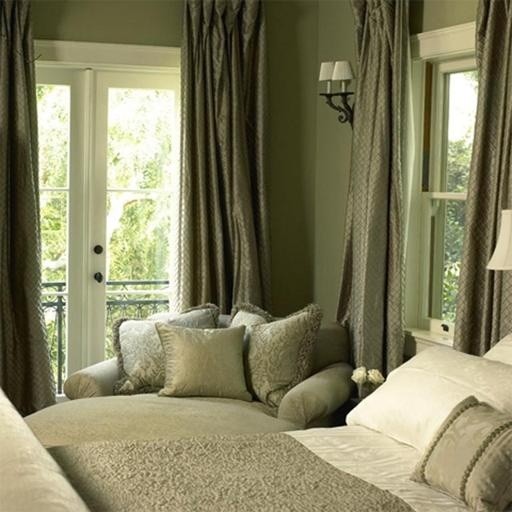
[352,367,383,385]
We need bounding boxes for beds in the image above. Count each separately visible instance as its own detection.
[47,424,478,512]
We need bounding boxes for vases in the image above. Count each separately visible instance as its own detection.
[357,384,380,399]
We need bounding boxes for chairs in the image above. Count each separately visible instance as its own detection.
[20,315,354,449]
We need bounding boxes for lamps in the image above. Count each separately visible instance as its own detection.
[486,210,512,270]
[318,60,356,130]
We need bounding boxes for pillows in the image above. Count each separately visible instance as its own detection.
[483,334,512,364]
[0,392,89,512]
[113,302,321,407]
[345,346,512,451]
[410,395,512,512]
